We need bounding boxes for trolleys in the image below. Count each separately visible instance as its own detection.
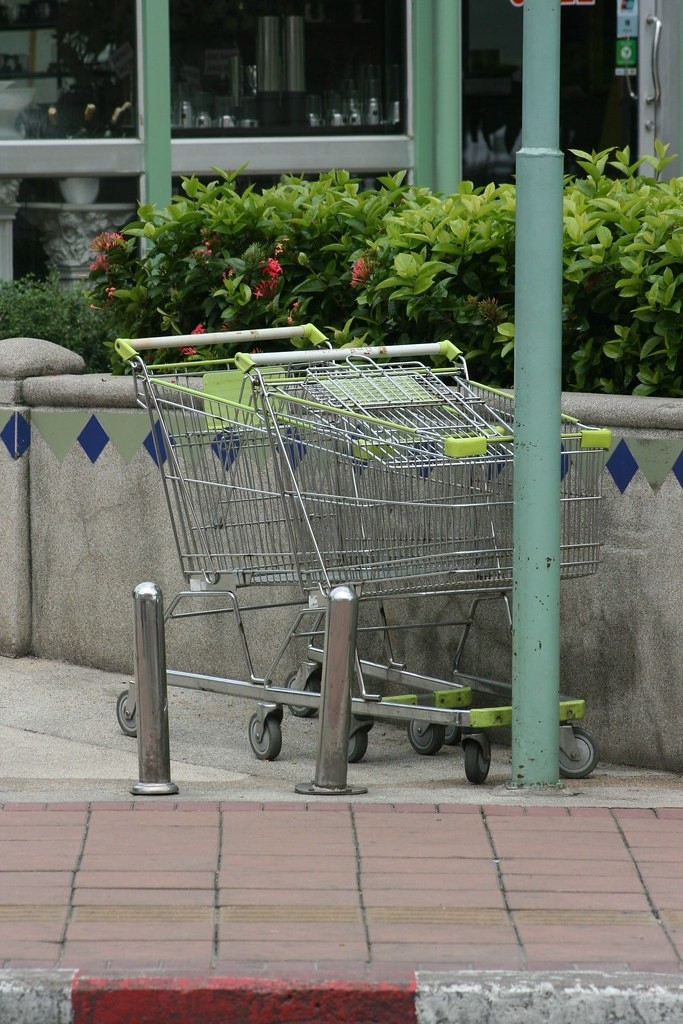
[115,323,612,783]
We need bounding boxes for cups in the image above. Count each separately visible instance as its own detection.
[309,98,381,128]
[283,91,306,124]
[257,91,280,126]
[178,101,258,128]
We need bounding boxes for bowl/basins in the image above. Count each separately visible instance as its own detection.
[0,88,38,139]
[58,178,100,204]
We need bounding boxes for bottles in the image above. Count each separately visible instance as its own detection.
[345,79,358,101]
[46,101,132,138]
[386,100,400,124]
